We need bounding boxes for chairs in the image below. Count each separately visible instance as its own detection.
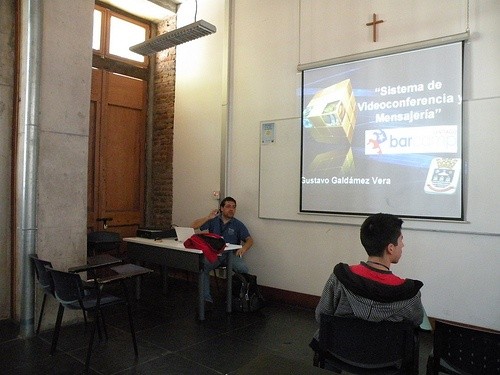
[426,319,500,375]
[309,312,426,375]
[28,232,138,375]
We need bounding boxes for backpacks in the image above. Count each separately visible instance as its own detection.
[232,271,259,312]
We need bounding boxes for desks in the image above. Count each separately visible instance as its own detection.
[123,236,242,323]
[89,240,122,244]
[68,253,153,284]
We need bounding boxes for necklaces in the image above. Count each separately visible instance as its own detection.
[366,261,390,271]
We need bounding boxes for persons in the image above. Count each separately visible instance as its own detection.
[308,214,425,355]
[190,197,253,311]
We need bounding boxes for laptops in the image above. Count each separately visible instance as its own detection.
[175,227,195,242]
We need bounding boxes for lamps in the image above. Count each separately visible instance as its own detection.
[129,0,216,57]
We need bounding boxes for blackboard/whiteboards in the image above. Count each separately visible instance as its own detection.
[258,96,500,237]
